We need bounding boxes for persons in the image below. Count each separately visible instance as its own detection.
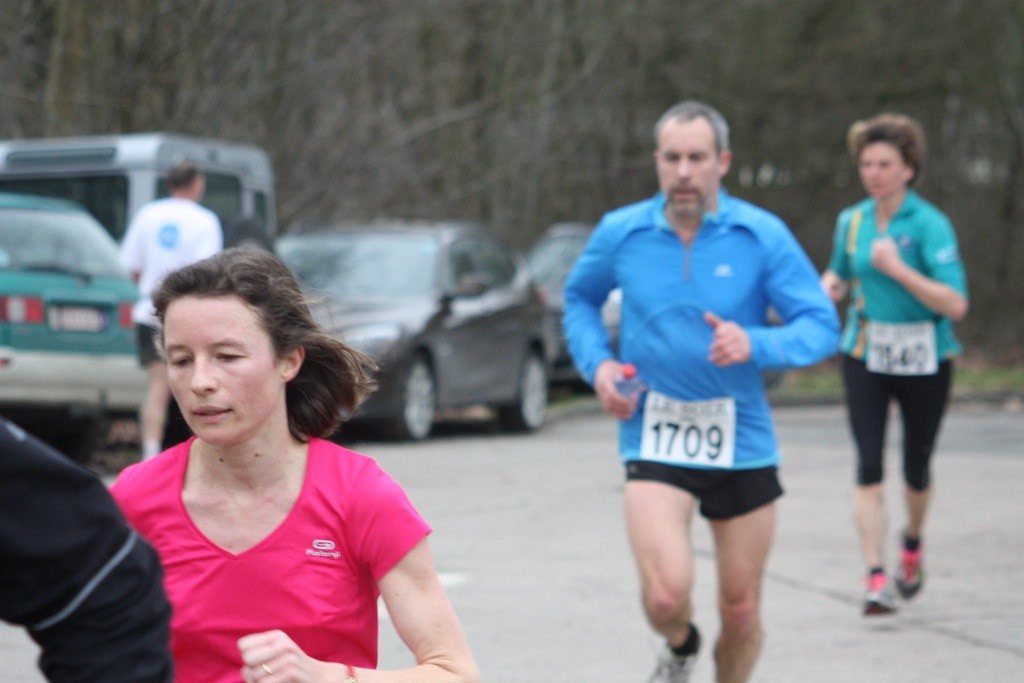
[121,162,223,462]
[0,247,484,683]
[561,100,841,683]
[820,114,970,614]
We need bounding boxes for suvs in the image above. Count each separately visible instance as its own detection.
[0,129,282,256]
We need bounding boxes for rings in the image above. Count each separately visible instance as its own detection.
[261,662,272,675]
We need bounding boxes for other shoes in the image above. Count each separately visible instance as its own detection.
[895,543,922,600]
[862,581,896,613]
[644,634,703,683]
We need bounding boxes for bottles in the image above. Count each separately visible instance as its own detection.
[614,364,641,406]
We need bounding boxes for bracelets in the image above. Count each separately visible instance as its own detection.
[342,663,359,683]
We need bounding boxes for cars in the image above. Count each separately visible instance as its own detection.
[0,192,149,468]
[270,218,553,445]
[525,220,791,387]
